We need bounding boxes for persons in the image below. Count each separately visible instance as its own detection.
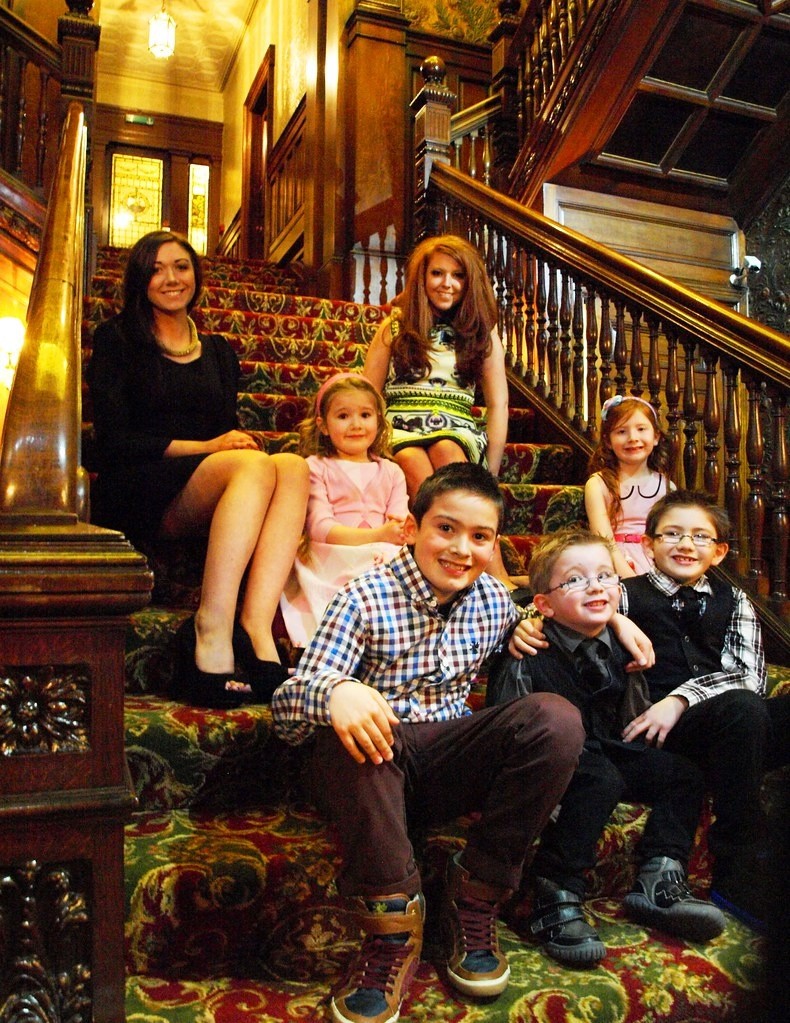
[271,463,585,1022]
[87,231,309,708]
[483,524,726,961]
[585,394,678,580]
[509,487,790,932]
[280,373,413,647]
[361,235,525,601]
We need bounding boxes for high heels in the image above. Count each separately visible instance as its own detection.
[168,612,246,709]
[234,621,292,703]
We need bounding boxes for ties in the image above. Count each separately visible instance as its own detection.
[676,585,703,622]
[582,640,611,691]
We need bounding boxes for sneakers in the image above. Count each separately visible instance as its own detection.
[436,849,512,997]
[623,856,726,940]
[327,891,427,1023]
[526,880,607,962]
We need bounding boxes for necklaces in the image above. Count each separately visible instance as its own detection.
[156,316,197,357]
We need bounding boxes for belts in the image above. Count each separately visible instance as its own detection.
[614,534,643,543]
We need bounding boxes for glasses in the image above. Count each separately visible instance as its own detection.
[543,571,619,594]
[652,531,725,546]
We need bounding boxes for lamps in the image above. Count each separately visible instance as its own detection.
[148,0,176,57]
